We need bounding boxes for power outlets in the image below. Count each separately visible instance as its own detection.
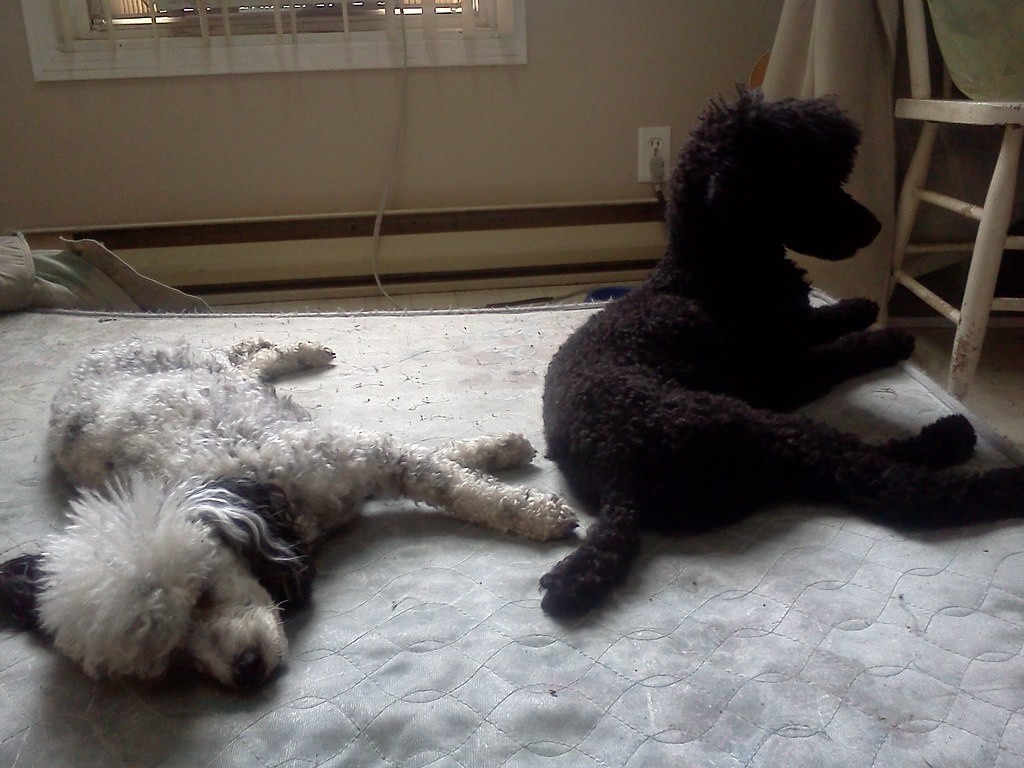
[637,125,671,184]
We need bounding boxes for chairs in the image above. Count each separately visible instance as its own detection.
[889,0,1024,401]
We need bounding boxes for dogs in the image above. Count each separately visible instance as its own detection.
[540,101,1024,616]
[38,334,578,692]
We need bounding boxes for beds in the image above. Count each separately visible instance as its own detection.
[0,286,1024,768]
